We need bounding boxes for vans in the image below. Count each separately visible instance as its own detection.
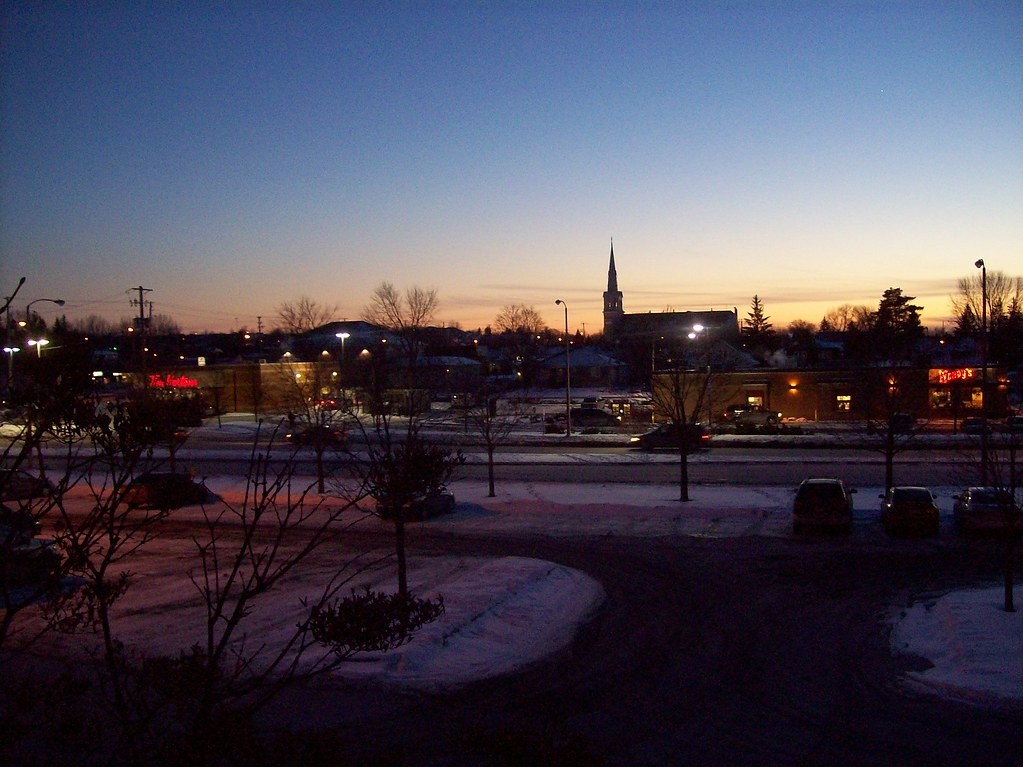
[722,404,778,426]
[571,408,622,429]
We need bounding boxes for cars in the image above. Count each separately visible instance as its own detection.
[892,412,915,433]
[878,487,940,531]
[1002,413,1023,432]
[0,466,23,499]
[952,485,1023,533]
[118,471,194,508]
[286,424,350,448]
[961,418,993,434]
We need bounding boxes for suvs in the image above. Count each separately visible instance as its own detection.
[791,477,858,531]
[630,420,709,453]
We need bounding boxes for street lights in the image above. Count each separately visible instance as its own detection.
[335,332,350,366]
[25,298,67,338]
[3,345,21,394]
[555,299,571,436]
[27,338,49,359]
[975,258,987,414]
